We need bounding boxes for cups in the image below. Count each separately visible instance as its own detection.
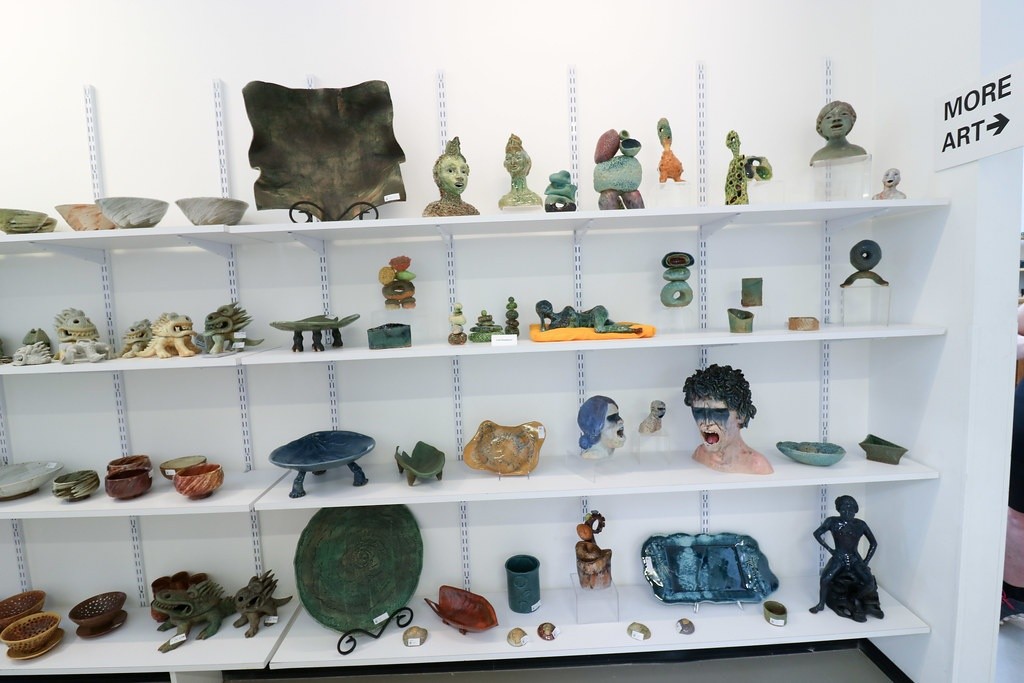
[763,600,787,627]
[504,554,541,614]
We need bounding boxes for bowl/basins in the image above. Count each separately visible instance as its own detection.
[789,316,819,330]
[107,454,153,482]
[0,207,57,233]
[160,455,207,480]
[0,460,65,501]
[55,204,116,231]
[104,467,153,500]
[52,470,100,501]
[94,196,169,229]
[777,441,844,466]
[172,463,225,500]
[175,197,249,226]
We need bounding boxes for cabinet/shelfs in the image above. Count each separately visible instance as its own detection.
[0,196,949,683]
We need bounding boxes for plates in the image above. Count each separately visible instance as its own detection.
[6,628,64,660]
[641,532,779,603]
[76,610,128,638]
[464,421,546,475]
